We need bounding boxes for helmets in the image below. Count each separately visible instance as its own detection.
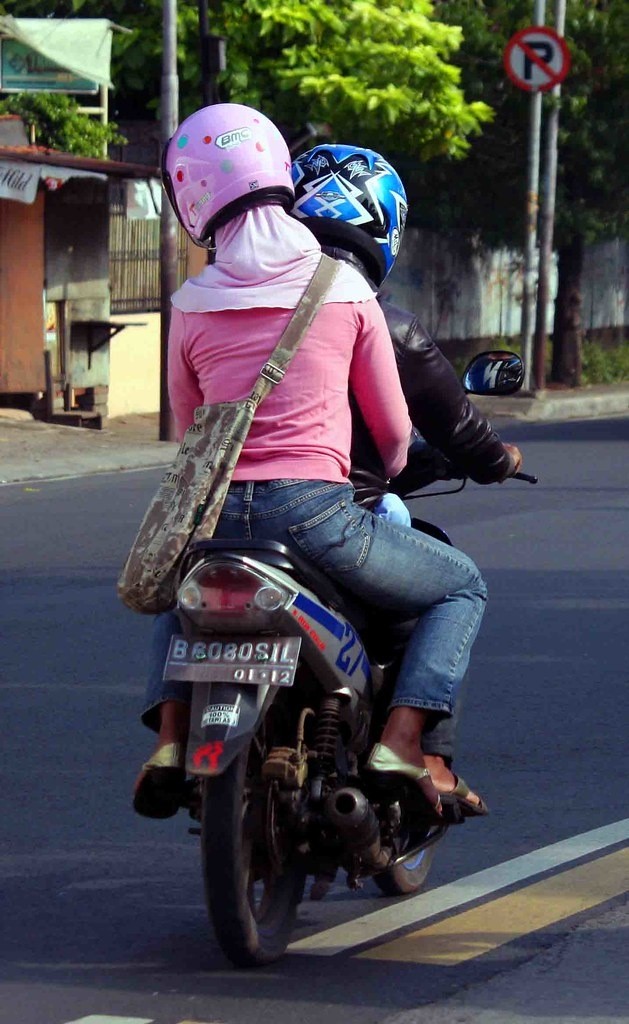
[290,142,409,288]
[161,103,295,250]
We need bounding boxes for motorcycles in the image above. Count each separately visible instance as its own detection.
[149,344,539,969]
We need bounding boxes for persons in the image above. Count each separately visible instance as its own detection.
[129,101,523,824]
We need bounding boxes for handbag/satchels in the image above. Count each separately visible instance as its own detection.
[117,255,339,616]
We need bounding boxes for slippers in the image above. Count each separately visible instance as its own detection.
[438,769,490,819]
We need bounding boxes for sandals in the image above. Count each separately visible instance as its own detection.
[365,745,446,823]
[133,742,188,819]
